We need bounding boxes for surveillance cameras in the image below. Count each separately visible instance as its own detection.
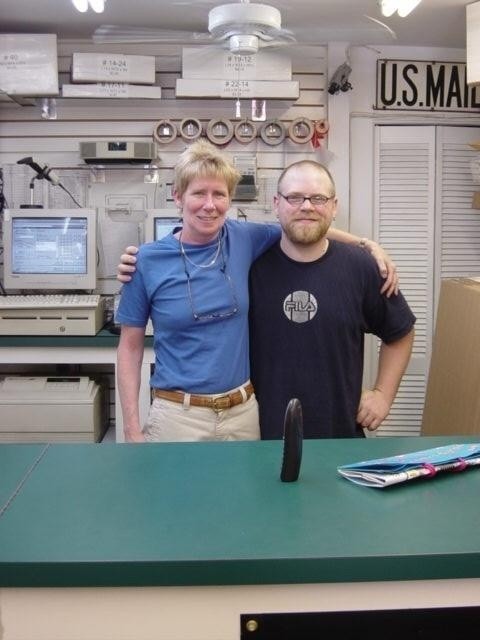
[328,62,352,95]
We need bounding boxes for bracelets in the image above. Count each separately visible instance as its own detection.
[359,237,369,249]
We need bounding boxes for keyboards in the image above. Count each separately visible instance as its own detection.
[0,294,100,309]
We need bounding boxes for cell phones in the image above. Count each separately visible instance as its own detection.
[280,398,304,482]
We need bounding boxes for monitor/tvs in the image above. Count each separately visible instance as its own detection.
[144,207,238,243]
[4,208,96,290]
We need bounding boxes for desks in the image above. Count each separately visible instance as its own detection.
[0,328,157,442]
[0,435,479,638]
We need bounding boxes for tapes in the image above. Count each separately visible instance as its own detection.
[154,116,329,145]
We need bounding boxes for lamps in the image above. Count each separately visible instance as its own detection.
[381,0,420,18]
[73,1,105,15]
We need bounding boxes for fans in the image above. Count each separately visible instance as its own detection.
[91,0,396,117]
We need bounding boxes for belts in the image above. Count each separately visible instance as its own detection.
[151,384,254,414]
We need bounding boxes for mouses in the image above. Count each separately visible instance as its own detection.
[109,325,121,335]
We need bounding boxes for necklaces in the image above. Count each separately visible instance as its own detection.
[180,226,221,269]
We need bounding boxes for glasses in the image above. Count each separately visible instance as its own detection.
[188,274,240,322]
[278,192,337,205]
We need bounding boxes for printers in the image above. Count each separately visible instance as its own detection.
[0,376,110,443]
